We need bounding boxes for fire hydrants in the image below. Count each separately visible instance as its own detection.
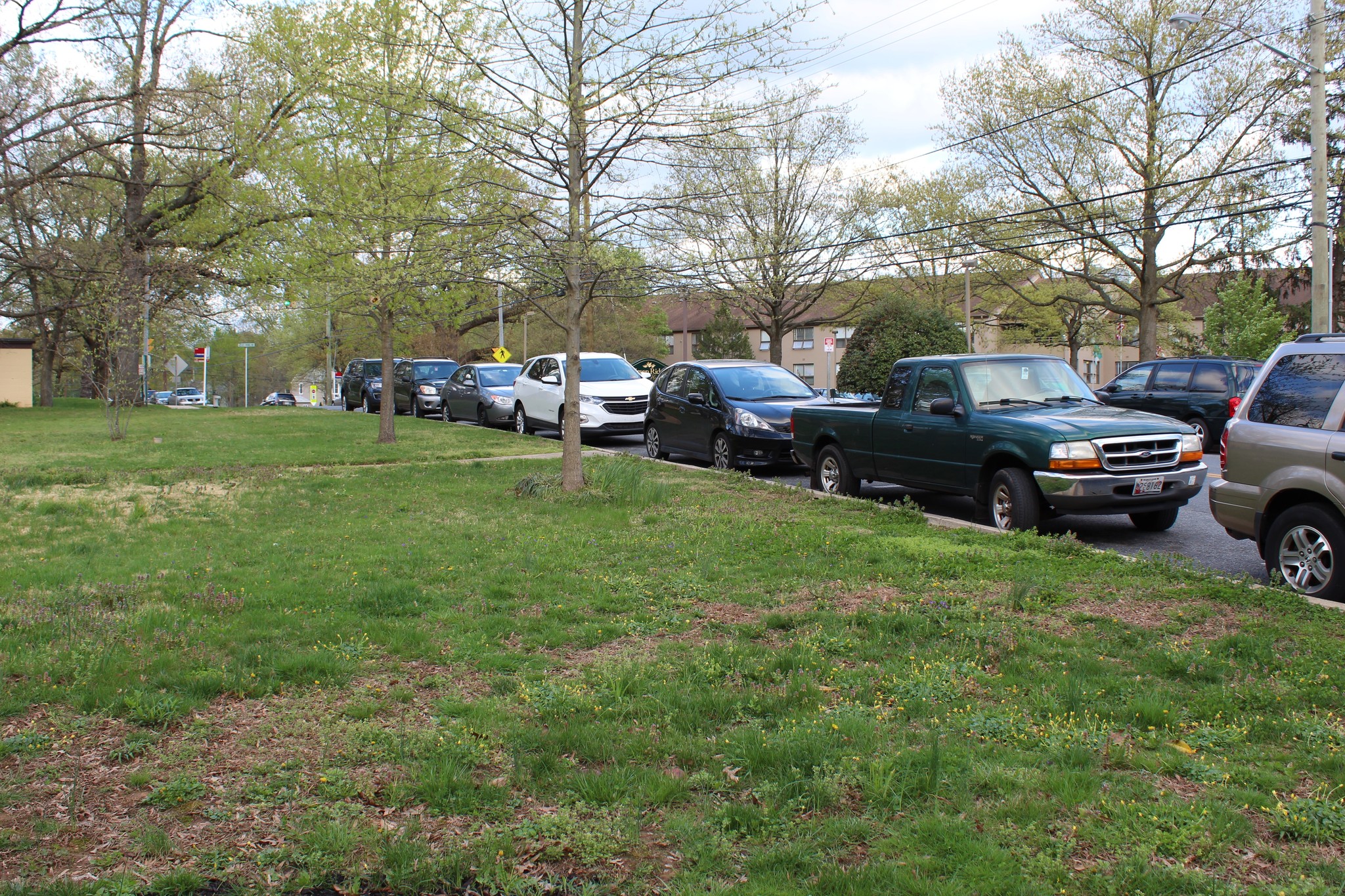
[212,390,222,408]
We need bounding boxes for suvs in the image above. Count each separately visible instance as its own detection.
[393,356,474,419]
[1091,354,1264,455]
[340,356,408,414]
[259,392,297,407]
[1208,330,1345,603]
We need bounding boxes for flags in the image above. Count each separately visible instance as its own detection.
[1115,314,1125,345]
[1156,340,1166,359]
[1089,326,1102,366]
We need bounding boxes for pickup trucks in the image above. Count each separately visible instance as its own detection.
[787,353,1202,537]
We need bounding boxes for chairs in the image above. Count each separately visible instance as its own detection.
[919,381,955,402]
[740,376,764,398]
[474,374,489,386]
[415,367,422,379]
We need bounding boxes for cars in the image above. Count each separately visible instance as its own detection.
[140,390,157,403]
[167,386,207,406]
[438,362,525,428]
[643,358,881,474]
[512,351,655,445]
[331,392,342,406]
[149,391,173,405]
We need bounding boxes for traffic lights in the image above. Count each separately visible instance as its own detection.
[147,338,155,352]
[370,289,378,303]
[283,284,292,306]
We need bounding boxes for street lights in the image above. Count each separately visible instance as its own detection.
[832,331,838,389]
[523,311,535,366]
[962,259,978,353]
[678,287,688,362]
[1166,13,1332,334]
